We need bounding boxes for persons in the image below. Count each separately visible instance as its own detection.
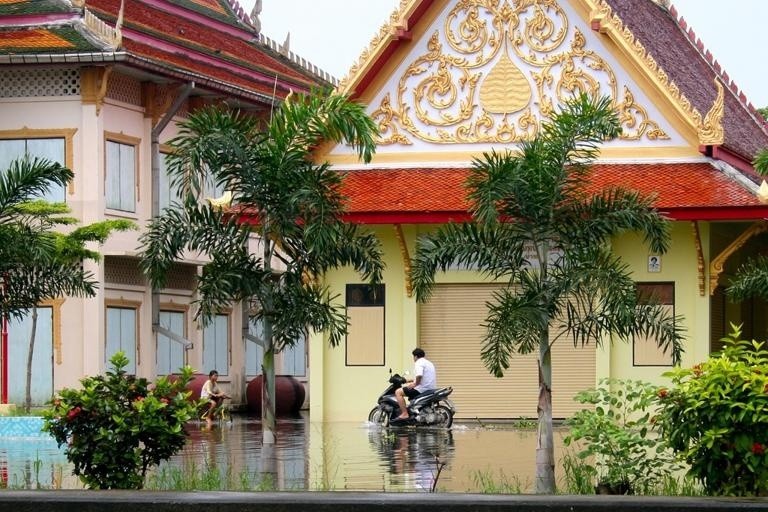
[392,348,435,421]
[201,370,225,423]
[649,257,659,271]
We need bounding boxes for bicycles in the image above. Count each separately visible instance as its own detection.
[196,394,233,423]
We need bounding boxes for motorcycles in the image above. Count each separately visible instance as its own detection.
[368,368,457,429]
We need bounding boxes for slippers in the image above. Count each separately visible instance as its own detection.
[392,416,409,422]
